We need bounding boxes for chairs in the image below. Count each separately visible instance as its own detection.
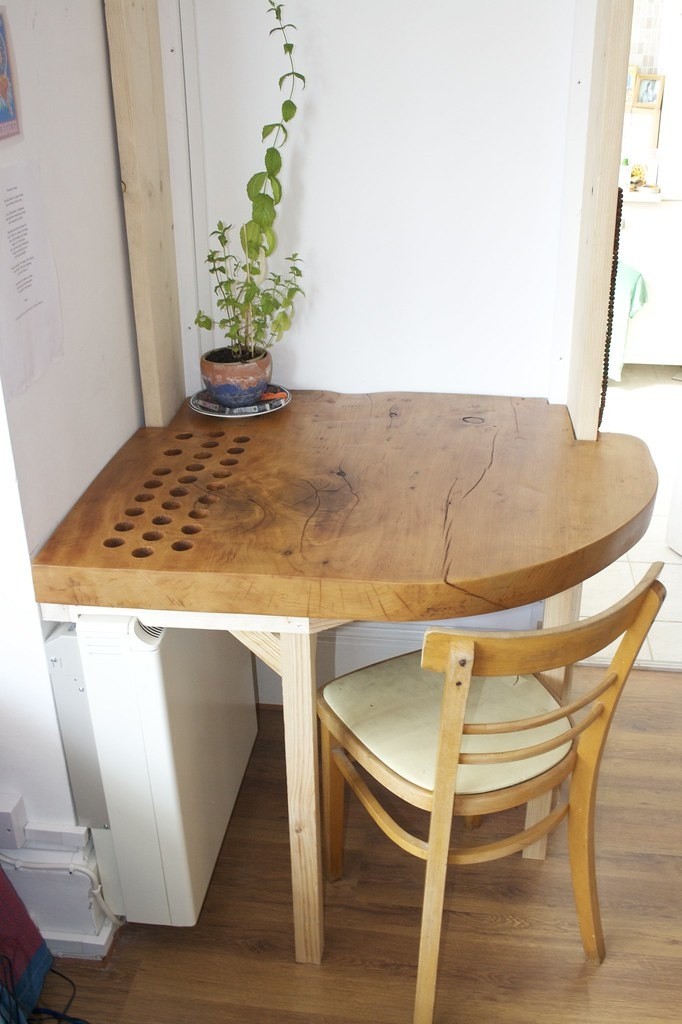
[313,558,664,1023]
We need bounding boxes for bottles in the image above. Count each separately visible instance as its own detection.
[618,159,631,200]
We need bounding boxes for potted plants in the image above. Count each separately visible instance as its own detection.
[191,0,307,419]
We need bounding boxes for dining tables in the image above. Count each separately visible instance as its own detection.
[33,366,663,970]
